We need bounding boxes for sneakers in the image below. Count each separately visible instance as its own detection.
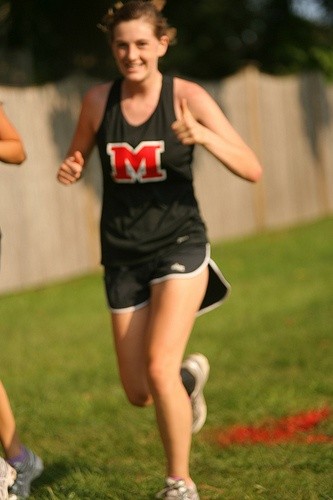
[179,351,210,437]
[5,448,45,500]
[0,457,18,500]
[155,477,201,499]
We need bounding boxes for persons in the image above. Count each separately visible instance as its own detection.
[0,108,42,500]
[57,0,263,499]
